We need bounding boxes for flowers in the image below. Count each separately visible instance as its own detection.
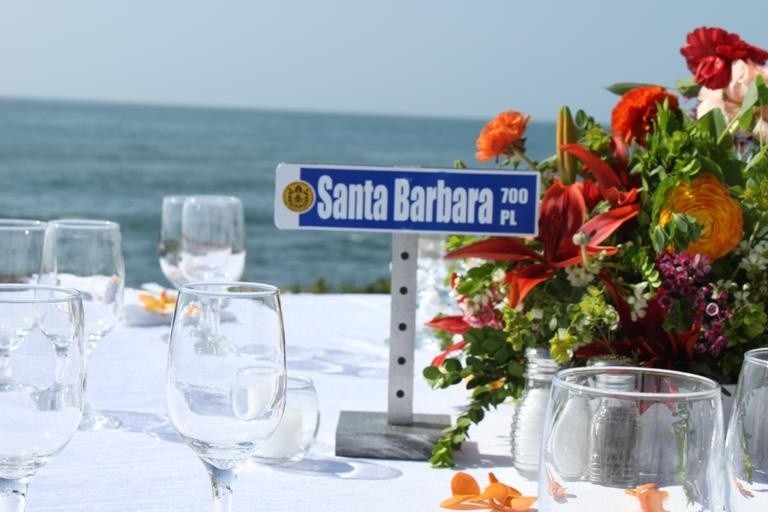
[423,26,768,470]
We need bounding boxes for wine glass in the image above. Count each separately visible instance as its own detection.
[157,193,247,361]
[34,218,128,430]
[0,282,95,512]
[159,280,290,512]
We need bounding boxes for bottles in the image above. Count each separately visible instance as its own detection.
[510,346,566,486]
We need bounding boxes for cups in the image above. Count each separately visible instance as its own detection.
[538,348,768,512]
[226,368,322,468]
[0,218,57,282]
